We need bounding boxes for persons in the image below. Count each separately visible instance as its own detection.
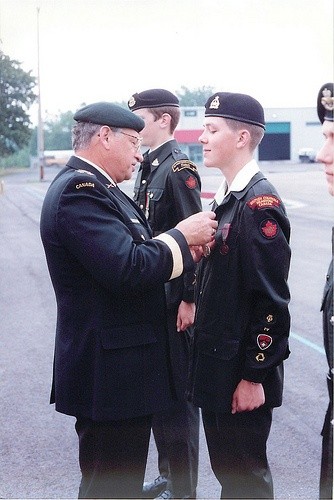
[40,102,219,499]
[316,83,334,500]
[128,89,203,500]
[202,90,292,500]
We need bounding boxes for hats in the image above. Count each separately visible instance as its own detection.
[317,84,334,123]
[128,88,181,111]
[203,92,268,129]
[74,101,146,131]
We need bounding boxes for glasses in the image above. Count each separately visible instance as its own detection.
[113,129,145,146]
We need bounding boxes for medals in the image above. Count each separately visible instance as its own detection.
[220,245,230,256]
[203,246,212,258]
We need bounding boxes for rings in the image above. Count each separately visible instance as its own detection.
[211,227,216,238]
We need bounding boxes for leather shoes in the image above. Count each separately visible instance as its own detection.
[142,475,171,497]
[154,489,177,500]
